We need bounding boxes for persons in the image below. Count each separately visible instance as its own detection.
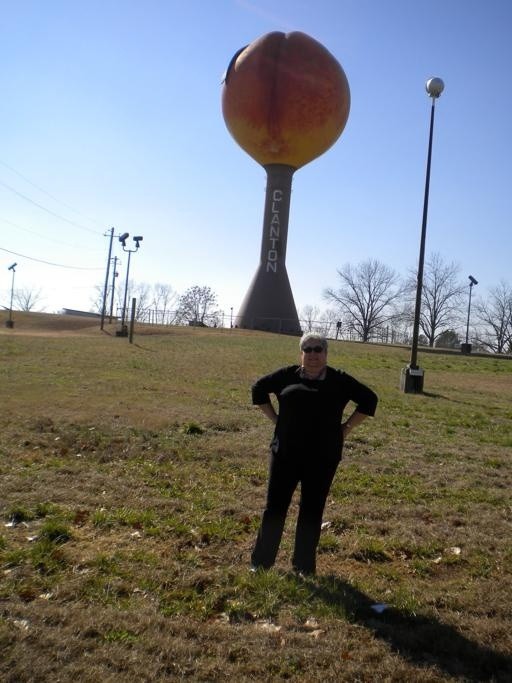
[251,330,378,578]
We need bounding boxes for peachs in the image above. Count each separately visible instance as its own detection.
[222,31,350,169]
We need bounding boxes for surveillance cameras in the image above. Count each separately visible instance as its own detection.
[8,262,17,271]
[119,233,129,243]
[133,236,143,241]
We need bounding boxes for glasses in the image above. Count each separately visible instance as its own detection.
[302,345,323,353]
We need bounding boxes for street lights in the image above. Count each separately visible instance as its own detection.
[116,233,143,336]
[230,306,234,328]
[402,75,445,395]
[461,275,478,354]
[5,262,17,328]
